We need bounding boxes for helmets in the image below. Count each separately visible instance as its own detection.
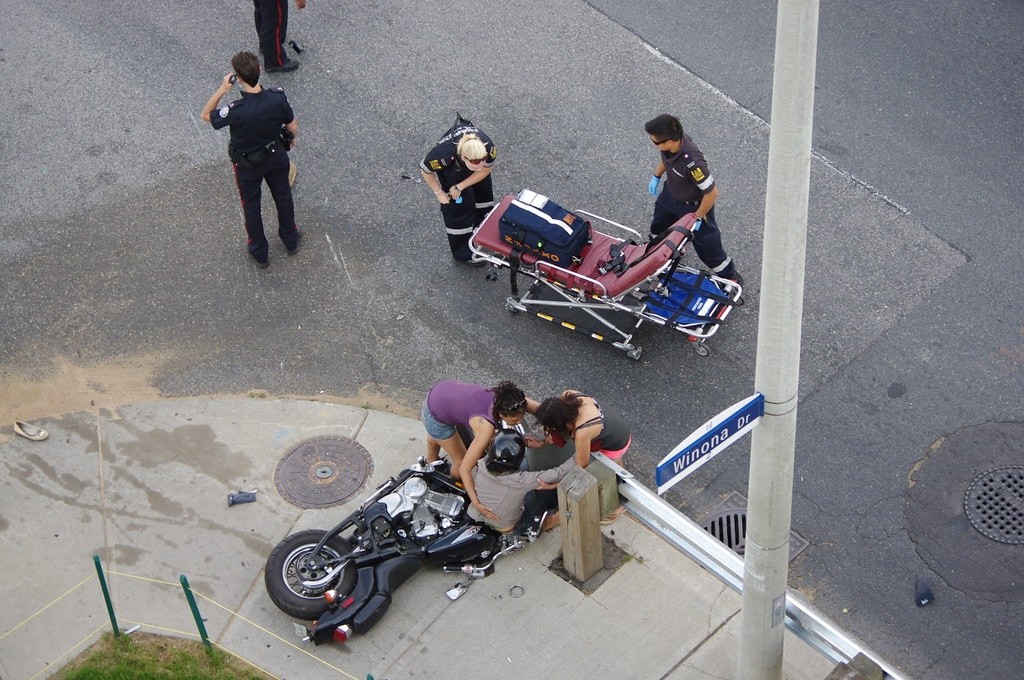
[489,429,528,468]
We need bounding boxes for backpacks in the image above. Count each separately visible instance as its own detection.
[499,188,591,267]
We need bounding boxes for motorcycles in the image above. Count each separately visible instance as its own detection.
[263,456,549,646]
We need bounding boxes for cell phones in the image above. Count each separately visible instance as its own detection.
[229,74,237,85]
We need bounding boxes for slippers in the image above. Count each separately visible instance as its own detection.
[599,507,627,525]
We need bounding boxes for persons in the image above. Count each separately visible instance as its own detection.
[421,381,544,523]
[529,389,632,524]
[202,52,303,267]
[253,0,306,73]
[419,110,497,267]
[644,114,745,295]
[467,428,577,542]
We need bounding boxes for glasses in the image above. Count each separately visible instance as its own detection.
[464,153,488,164]
[650,136,671,146]
[509,398,527,412]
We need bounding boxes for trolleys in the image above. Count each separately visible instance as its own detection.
[467,195,742,361]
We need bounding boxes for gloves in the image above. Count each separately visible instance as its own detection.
[695,218,701,231]
[648,174,661,196]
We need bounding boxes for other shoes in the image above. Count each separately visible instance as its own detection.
[733,274,744,291]
[264,57,297,71]
[453,252,487,267]
[287,223,302,254]
[248,237,268,268]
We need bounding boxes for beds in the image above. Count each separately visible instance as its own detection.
[468,194,742,361]
